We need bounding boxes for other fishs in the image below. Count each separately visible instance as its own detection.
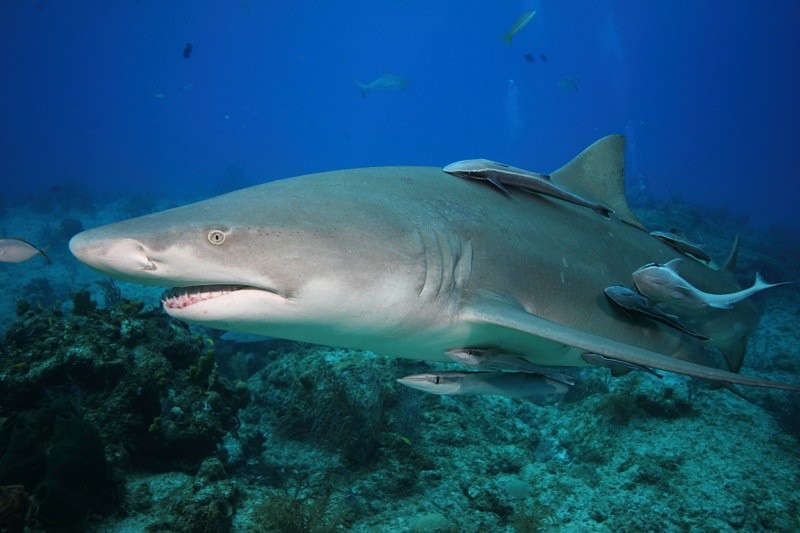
[397,348,578,397]
[604,259,796,342]
[1,10,537,264]
